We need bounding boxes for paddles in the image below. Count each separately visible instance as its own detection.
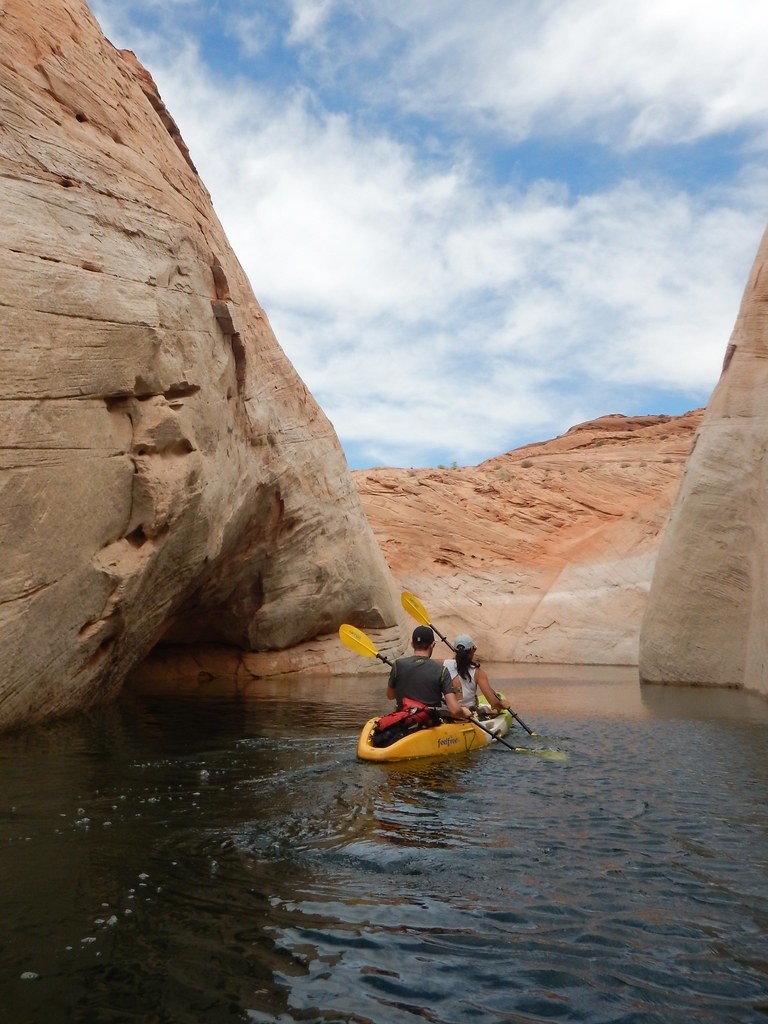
[399,590,537,736]
[338,622,567,761]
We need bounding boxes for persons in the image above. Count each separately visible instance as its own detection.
[386,625,473,731]
[434,634,510,717]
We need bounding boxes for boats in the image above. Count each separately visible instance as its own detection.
[356,692,513,765]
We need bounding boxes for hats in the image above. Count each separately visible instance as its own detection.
[454,634,475,650]
[412,625,434,645]
